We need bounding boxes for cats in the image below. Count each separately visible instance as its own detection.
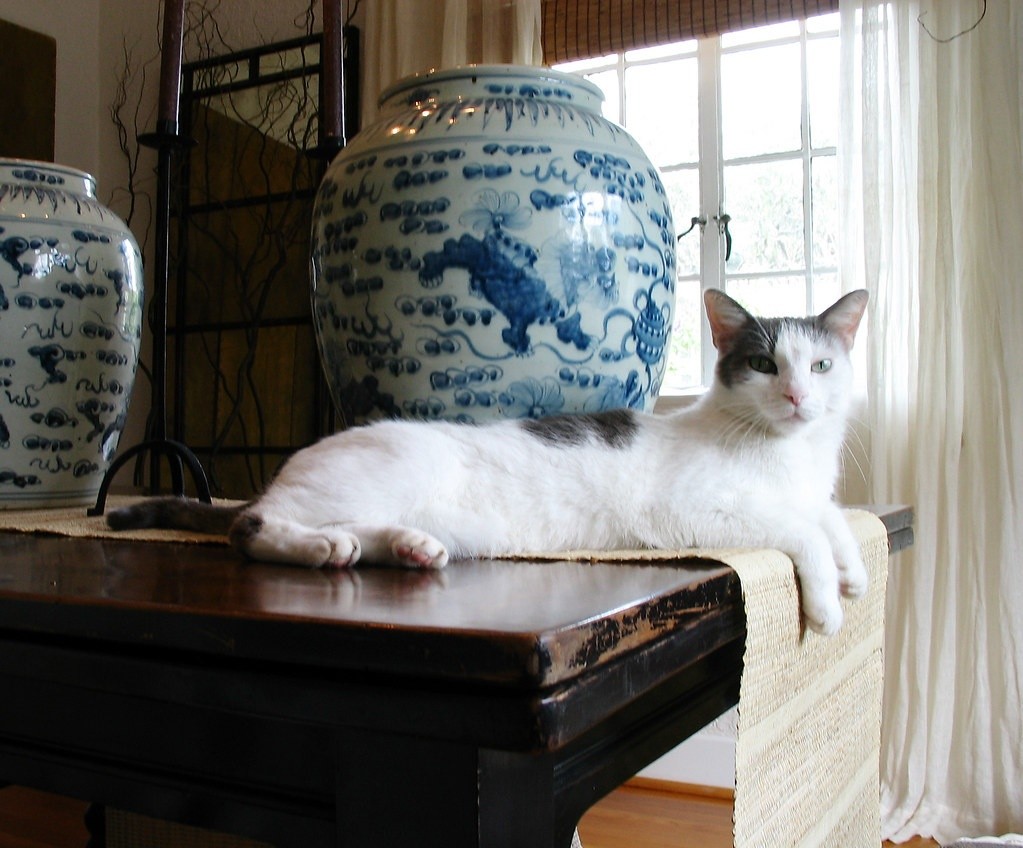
[105,289,870,636]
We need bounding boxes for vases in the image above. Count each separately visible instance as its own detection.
[308,64,675,430]
[0,157,144,508]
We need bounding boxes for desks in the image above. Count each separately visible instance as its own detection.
[0,493,916,848]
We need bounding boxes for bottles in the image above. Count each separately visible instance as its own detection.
[0,157,150,511]
[308,64,676,427]
